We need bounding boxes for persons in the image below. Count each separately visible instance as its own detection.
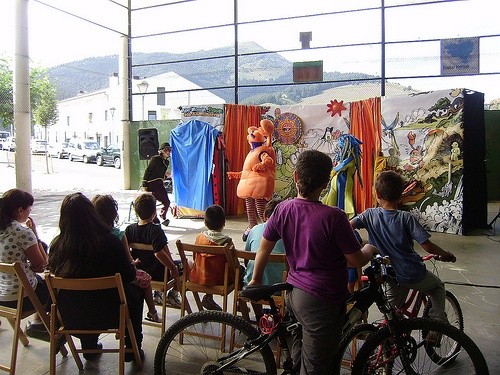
[0,188,52,342]
[321,134,362,221]
[125,194,194,323]
[90,194,162,322]
[244,209,286,331]
[243,150,376,375]
[190,205,251,314]
[142,142,177,226]
[46,193,144,362]
[349,172,455,375]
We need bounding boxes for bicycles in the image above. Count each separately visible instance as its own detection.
[154,253,491,375]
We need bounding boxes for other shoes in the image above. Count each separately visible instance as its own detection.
[123,349,144,362]
[202,295,223,310]
[166,290,181,308]
[26,322,50,341]
[153,291,164,305]
[83,342,103,359]
[427,330,442,347]
[237,302,252,313]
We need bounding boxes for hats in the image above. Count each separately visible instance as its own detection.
[159,143,173,150]
[246,119,273,143]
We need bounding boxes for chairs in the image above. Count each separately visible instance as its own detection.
[0,239,367,375]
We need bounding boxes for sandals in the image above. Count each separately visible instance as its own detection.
[144,311,162,323]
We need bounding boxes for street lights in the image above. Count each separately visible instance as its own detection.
[137,81,149,121]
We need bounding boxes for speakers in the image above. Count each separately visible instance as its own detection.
[138,128,159,160]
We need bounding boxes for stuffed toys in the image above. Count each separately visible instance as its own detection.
[227,119,276,242]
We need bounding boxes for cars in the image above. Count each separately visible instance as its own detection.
[0,131,122,169]
